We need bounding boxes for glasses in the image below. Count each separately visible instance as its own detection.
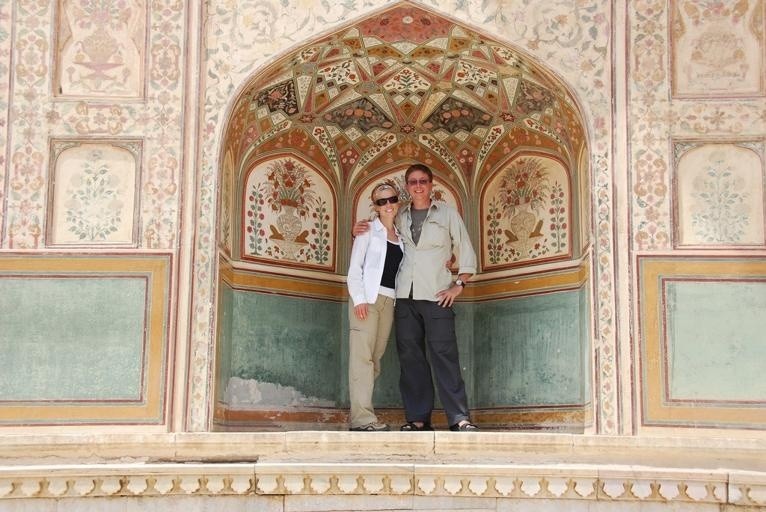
[373,192,399,206]
[408,177,431,185]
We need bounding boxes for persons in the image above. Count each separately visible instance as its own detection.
[344,182,404,434]
[351,162,482,433]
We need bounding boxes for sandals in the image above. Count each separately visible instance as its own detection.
[450,421,480,432]
[349,421,391,432]
[401,422,432,431]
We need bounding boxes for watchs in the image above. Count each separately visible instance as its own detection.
[455,279,467,290]
